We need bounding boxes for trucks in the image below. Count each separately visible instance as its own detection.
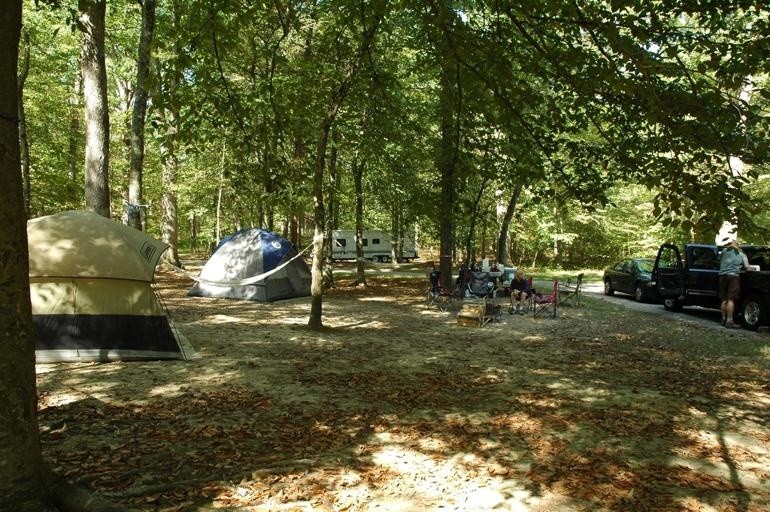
[310,230,420,262]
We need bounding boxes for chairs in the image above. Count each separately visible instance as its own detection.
[505,273,586,319]
[425,271,453,312]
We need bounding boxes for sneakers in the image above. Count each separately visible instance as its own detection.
[519,310,524,315]
[720,319,742,328]
[510,309,517,314]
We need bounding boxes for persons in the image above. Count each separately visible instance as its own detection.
[717,240,755,328]
[509,270,529,315]
[490,263,500,272]
[455,264,470,288]
[471,263,480,272]
[430,267,441,295]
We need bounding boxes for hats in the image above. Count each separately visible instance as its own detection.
[724,240,739,251]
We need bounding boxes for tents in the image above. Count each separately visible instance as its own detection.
[27,209,184,364]
[187,226,312,303]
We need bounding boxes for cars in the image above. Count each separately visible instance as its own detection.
[603,243,770,331]
